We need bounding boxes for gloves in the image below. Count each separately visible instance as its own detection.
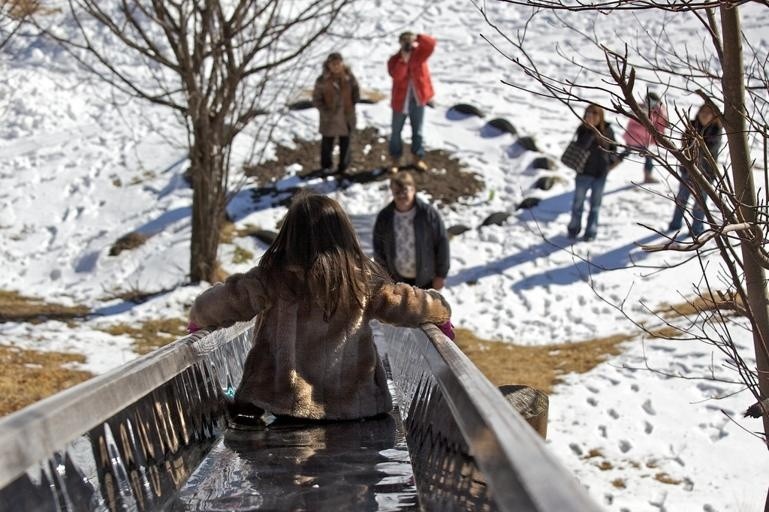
[186,320,204,337]
[436,316,457,344]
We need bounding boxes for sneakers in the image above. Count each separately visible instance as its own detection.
[584,234,594,241]
[568,230,576,240]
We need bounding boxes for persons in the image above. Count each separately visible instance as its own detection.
[666,101,724,237]
[566,104,619,245]
[370,169,452,295]
[384,30,439,174]
[184,192,458,424]
[311,53,360,179]
[616,90,672,185]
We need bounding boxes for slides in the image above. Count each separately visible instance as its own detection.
[0,315,606,511]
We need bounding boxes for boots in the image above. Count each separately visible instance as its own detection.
[644,172,660,183]
[413,155,427,171]
[391,157,400,174]
[612,158,621,168]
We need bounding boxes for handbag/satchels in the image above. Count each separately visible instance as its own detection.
[561,128,595,173]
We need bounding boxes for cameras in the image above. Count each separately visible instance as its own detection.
[403,40,412,50]
